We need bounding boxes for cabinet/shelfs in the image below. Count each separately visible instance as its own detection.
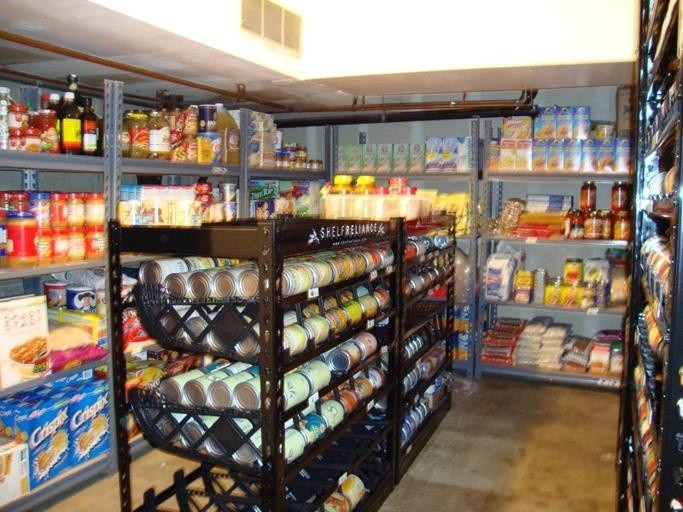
[0,1,683,512]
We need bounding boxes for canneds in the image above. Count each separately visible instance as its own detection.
[0,191,107,269]
[565,180,633,240]
[123,111,171,159]
[6,104,60,154]
[329,174,407,194]
[544,259,594,309]
[137,233,455,467]
[276,130,324,171]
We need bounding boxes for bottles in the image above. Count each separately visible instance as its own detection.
[0,73,97,154]
[570,180,633,239]
[324,174,431,221]
[533,247,631,309]
[121,100,240,168]
